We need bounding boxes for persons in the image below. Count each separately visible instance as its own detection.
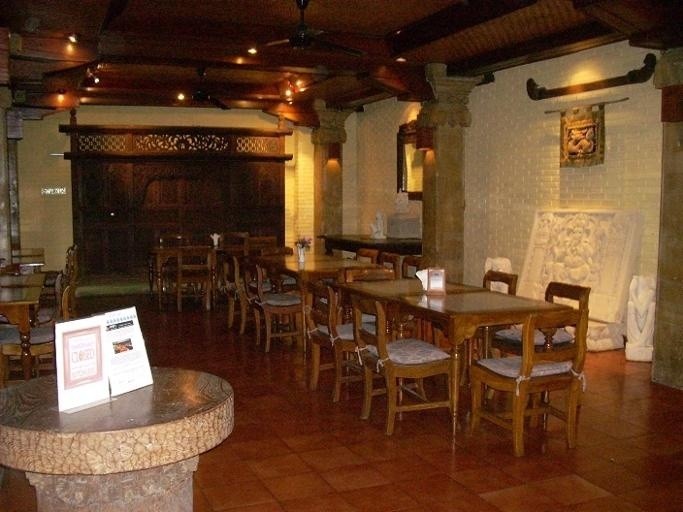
[545,212,612,287]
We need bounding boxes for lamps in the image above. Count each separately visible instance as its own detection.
[414,125,434,152]
[328,142,340,162]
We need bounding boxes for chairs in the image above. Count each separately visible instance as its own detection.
[238,264,302,352]
[469,310,588,457]
[0,245,80,387]
[350,295,453,435]
[492,281,591,428]
[217,250,272,333]
[151,233,423,312]
[459,271,518,387]
[306,282,378,402]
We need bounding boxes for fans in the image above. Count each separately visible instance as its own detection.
[263,0,370,58]
[187,68,230,111]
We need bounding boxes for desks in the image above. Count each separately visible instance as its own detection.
[0,366,233,512]
